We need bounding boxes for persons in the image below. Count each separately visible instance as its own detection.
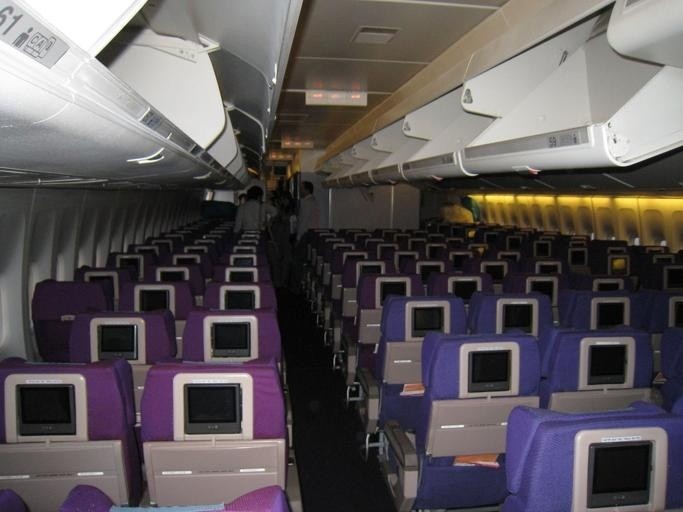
[232,181,320,294]
